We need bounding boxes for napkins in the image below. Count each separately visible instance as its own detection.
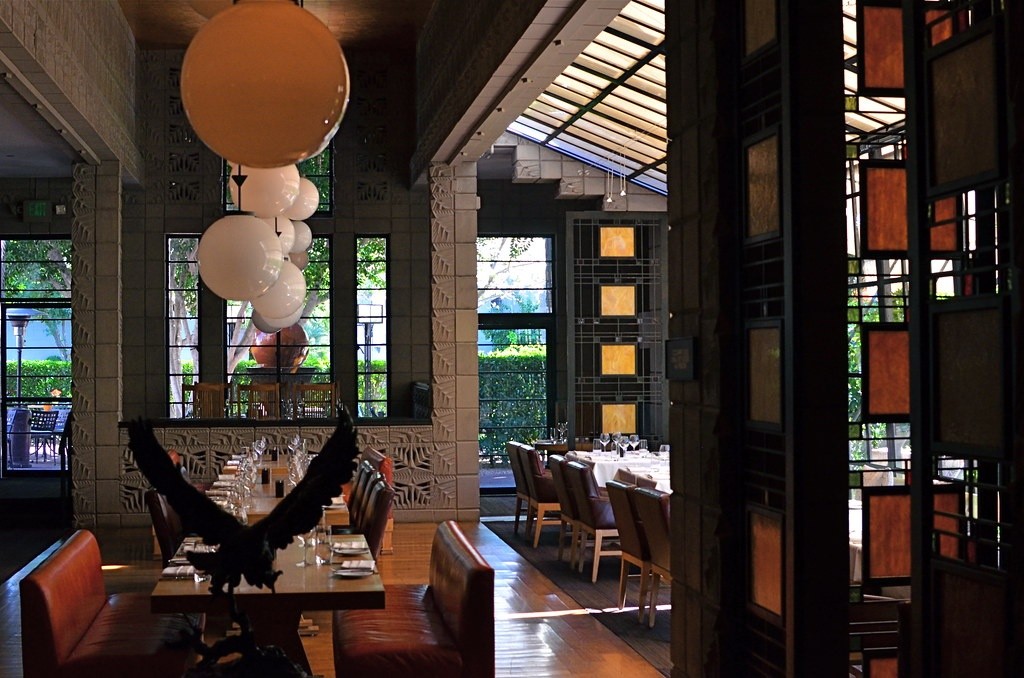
[161,567,203,576]
[334,541,367,549]
[183,545,208,553]
[341,560,375,570]
[205,455,242,507]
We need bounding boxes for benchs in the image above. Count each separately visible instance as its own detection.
[332,520,496,678]
[19,529,207,678]
[358,445,394,556]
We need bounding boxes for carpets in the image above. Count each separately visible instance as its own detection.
[479,495,675,678]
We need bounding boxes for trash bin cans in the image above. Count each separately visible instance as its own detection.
[9,410,32,466]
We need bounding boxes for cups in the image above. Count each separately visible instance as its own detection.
[550,428,558,440]
[592,438,601,454]
[315,525,333,565]
[640,440,647,458]
[650,452,659,472]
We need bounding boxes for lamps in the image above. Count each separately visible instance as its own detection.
[181,0,350,334]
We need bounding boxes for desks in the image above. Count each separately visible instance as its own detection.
[564,450,658,488]
[613,466,671,496]
[849,499,862,582]
[151,459,386,677]
[531,440,614,452]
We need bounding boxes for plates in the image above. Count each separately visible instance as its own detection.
[334,548,369,555]
[334,569,373,576]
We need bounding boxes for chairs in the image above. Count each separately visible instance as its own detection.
[147,464,194,570]
[7,405,72,467]
[333,457,394,568]
[181,382,337,418]
[507,439,674,628]
[856,465,894,500]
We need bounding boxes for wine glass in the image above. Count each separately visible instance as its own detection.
[228,434,326,565]
[659,445,669,469]
[557,422,566,441]
[600,432,639,459]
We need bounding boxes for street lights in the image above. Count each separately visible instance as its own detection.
[5,308,48,408]
